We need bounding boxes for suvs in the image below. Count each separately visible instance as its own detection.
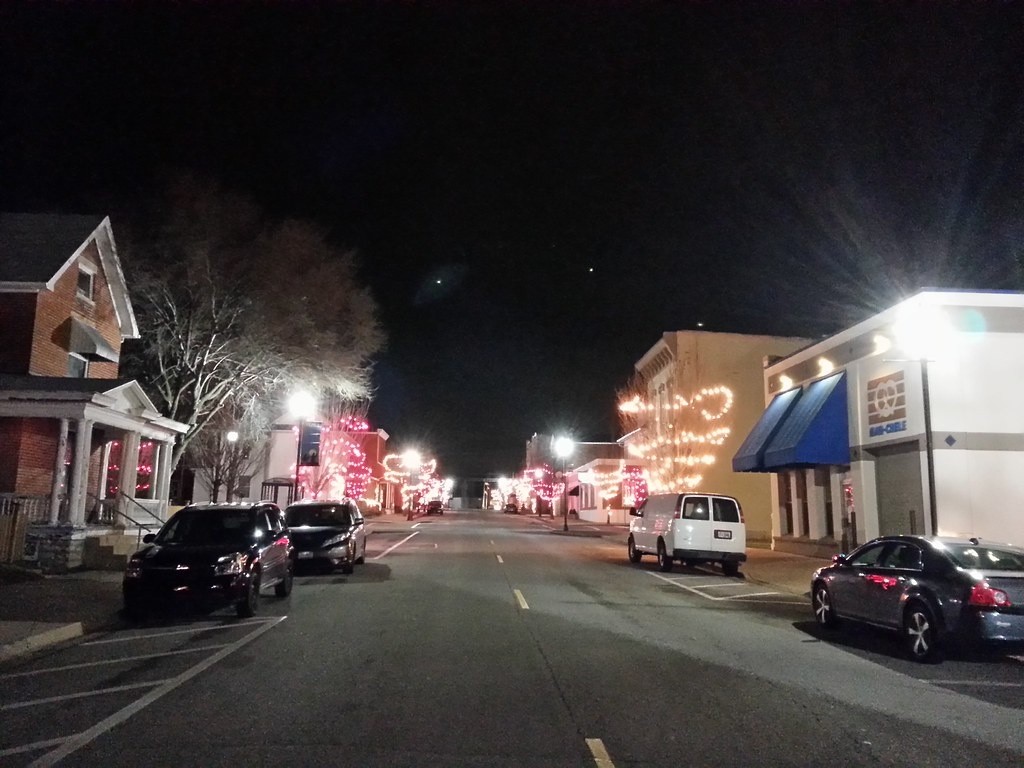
[122,500,295,621]
[284,496,367,574]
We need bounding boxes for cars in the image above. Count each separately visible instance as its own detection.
[504,504,518,514]
[427,501,444,516]
[810,533,1024,664]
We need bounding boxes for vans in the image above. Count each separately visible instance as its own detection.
[627,492,747,575]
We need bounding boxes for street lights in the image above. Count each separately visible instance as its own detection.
[554,436,575,531]
[891,307,948,536]
[402,450,419,521]
[287,389,316,502]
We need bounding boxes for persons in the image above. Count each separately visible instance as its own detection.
[607,504,612,524]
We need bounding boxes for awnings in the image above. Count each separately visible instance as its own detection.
[731,387,803,473]
[568,486,579,497]
[764,368,850,468]
[71,317,119,363]
